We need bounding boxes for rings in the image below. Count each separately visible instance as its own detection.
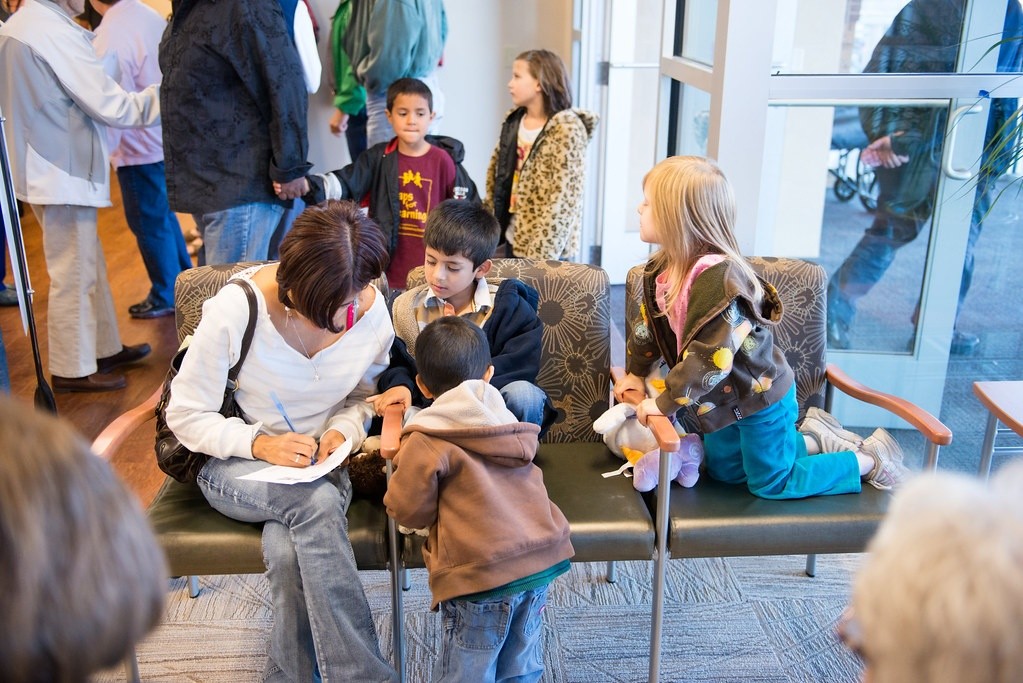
[295,454,299,462]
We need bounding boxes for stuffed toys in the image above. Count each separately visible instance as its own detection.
[594,356,704,491]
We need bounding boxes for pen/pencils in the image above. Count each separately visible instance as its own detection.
[270,391,319,463]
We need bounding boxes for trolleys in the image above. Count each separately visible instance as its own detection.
[830,104,882,213]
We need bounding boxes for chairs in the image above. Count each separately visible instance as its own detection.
[404,263,660,683]
[82,262,390,683]
[628,258,949,683]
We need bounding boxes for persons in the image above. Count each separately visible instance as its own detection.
[87,0,192,319]
[330,0,448,164]
[273,77,483,290]
[0,0,161,392]
[165,0,322,268]
[383,316,576,683]
[0,392,172,683]
[391,199,558,440]
[613,155,911,499]
[486,49,599,262]
[848,461,1023,683]
[827,0,1023,349]
[164,199,395,683]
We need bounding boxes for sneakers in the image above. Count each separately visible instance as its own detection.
[129,291,175,317]
[798,406,862,454]
[855,426,913,493]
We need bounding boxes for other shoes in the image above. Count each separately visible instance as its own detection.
[907,327,980,354]
[0,289,18,305]
[826,314,848,349]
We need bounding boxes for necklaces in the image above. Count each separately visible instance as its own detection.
[285,307,327,383]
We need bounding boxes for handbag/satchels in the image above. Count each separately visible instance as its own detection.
[154,278,258,484]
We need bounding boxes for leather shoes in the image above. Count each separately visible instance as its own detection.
[96,342,151,370]
[51,372,126,393]
